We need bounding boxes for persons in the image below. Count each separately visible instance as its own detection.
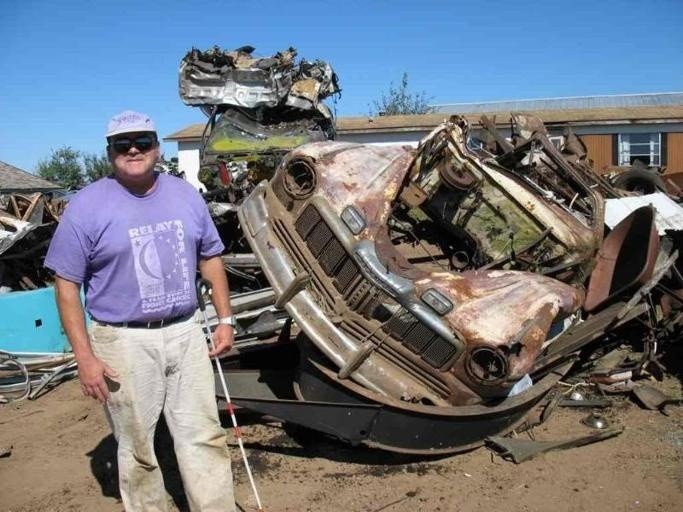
[41,109,250,512]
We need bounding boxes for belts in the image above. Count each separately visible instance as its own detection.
[89,313,191,330]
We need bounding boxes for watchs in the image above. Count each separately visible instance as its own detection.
[219,314,237,327]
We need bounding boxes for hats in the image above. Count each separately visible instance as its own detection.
[107,112,157,138]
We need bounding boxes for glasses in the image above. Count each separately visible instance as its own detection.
[107,135,159,154]
